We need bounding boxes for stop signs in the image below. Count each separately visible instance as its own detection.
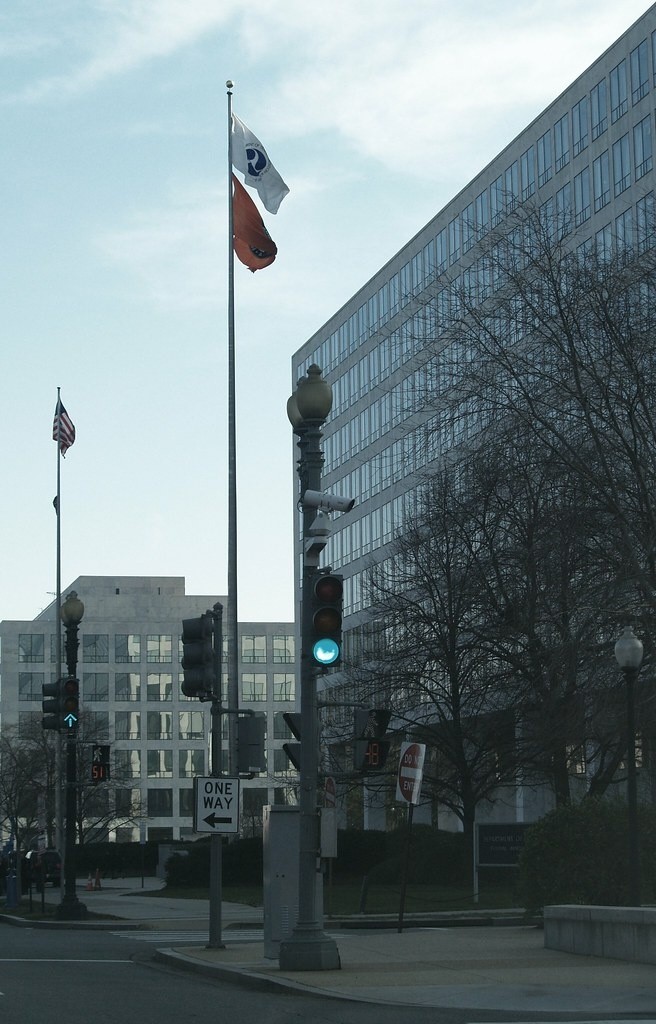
[324,777,336,810]
[398,744,421,804]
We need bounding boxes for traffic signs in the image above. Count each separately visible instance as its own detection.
[195,777,240,833]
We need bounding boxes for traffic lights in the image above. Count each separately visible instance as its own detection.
[282,710,302,741]
[302,573,344,668]
[354,737,391,772]
[92,745,111,781]
[284,743,302,772]
[180,613,221,699]
[354,707,393,742]
[41,680,59,730]
[58,676,80,730]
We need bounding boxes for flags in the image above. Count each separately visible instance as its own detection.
[227,112,290,215]
[228,171,277,274]
[52,397,75,458]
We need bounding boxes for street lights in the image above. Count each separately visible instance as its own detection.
[279,362,341,973]
[614,625,645,908]
[57,590,89,916]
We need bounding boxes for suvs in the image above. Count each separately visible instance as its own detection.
[24,848,61,888]
